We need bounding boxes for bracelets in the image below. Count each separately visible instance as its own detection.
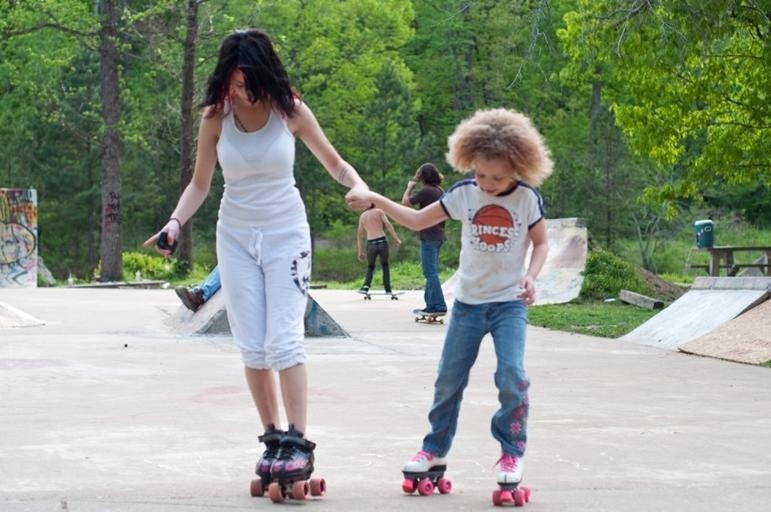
[167,217,182,232]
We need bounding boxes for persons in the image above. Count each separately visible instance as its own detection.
[354,193,401,296]
[399,162,449,315]
[173,261,223,315]
[343,104,553,505]
[140,27,374,503]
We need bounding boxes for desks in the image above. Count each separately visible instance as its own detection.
[693,243,770,278]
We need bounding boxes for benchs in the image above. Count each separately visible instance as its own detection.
[690,261,770,277]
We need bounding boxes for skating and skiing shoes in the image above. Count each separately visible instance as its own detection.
[251,424,325,502]
[401,451,451,495]
[493,454,529,506]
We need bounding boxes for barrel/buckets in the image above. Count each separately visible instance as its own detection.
[694,218,715,250]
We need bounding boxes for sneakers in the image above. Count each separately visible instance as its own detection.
[359,284,369,292]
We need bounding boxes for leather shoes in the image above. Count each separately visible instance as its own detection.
[175,288,201,312]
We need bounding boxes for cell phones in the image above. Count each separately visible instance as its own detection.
[157,232,178,255]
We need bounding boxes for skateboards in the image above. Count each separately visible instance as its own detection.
[360,290,404,300]
[413,310,446,325]
